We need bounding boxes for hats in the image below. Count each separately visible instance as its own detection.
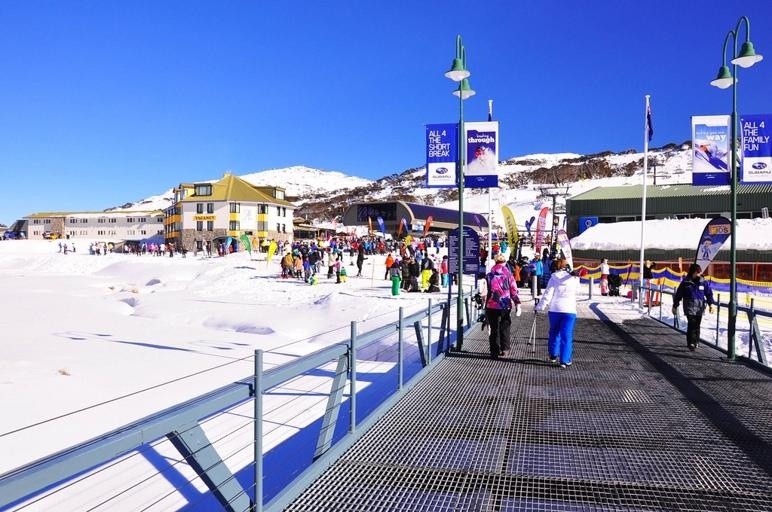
[534,252,541,256]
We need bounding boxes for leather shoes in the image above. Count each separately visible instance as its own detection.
[688,343,695,351]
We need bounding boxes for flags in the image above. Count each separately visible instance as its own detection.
[424,123,459,189]
[488,105,493,121]
[462,121,499,189]
[738,112,772,187]
[691,114,732,187]
[645,102,654,141]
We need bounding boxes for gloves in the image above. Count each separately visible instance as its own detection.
[709,304,715,313]
[515,303,522,316]
[672,307,677,315]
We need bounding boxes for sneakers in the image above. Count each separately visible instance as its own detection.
[549,356,556,363]
[502,348,511,355]
[559,360,572,368]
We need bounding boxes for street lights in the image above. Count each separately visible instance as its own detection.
[445,33,477,355]
[711,14,765,368]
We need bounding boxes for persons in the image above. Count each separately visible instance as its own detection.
[484,254,522,360]
[385,235,453,294]
[672,263,715,352]
[643,259,656,287]
[58,242,62,252]
[534,259,578,367]
[72,243,75,252]
[63,244,67,254]
[88,238,237,258]
[695,144,727,170]
[598,256,609,296]
[253,233,392,286]
[477,234,559,296]
